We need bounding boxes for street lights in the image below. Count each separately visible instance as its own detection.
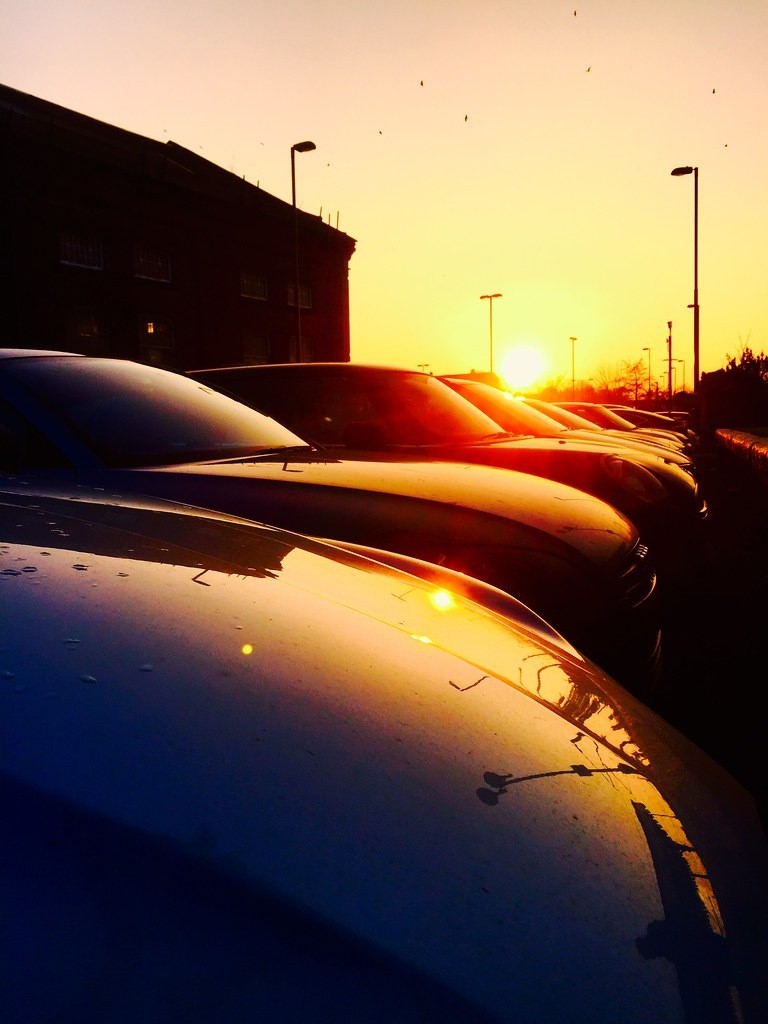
[288,138,315,364]
[569,337,579,401]
[481,294,504,373]
[640,317,687,403]
[671,165,701,431]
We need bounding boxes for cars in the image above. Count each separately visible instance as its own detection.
[0,345,767,1024]
